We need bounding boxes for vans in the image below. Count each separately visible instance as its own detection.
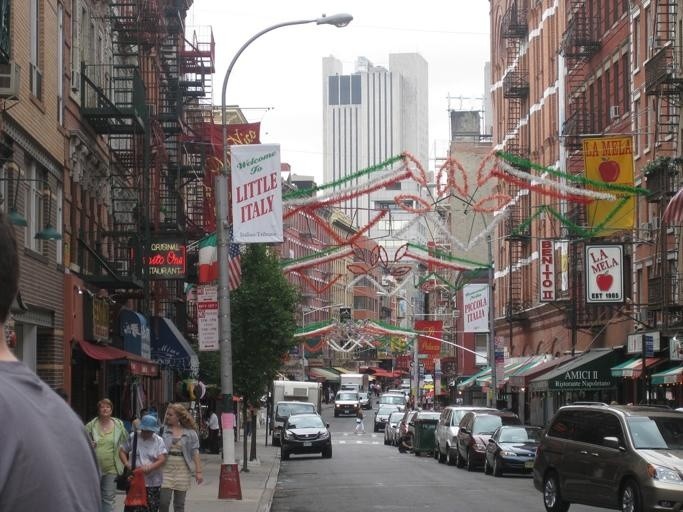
[532,401,683,512]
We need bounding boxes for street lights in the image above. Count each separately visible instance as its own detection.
[215,11,352,463]
[302,303,345,381]
[397,295,417,411]
[425,182,498,408]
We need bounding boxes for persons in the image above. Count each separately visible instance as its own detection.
[0,210,103,512]
[86,398,262,511]
[352,406,367,434]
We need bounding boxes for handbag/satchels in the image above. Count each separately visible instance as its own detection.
[114,466,132,491]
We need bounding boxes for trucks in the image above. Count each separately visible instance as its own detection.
[338,373,368,399]
[270,380,322,445]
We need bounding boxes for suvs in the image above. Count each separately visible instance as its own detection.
[278,412,332,460]
[334,391,362,417]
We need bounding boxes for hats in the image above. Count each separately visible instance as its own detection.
[135,415,161,433]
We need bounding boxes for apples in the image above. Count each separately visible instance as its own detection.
[598,156,620,182]
[596,269,613,291]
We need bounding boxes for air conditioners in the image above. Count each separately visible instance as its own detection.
[610,105,621,119]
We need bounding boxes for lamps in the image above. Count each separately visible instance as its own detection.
[0,156,27,226]
[0,178,61,240]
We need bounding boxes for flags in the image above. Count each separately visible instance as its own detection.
[199,231,219,284]
[226,228,242,291]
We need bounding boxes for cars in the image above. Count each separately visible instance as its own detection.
[358,392,372,409]
[374,389,545,476]
